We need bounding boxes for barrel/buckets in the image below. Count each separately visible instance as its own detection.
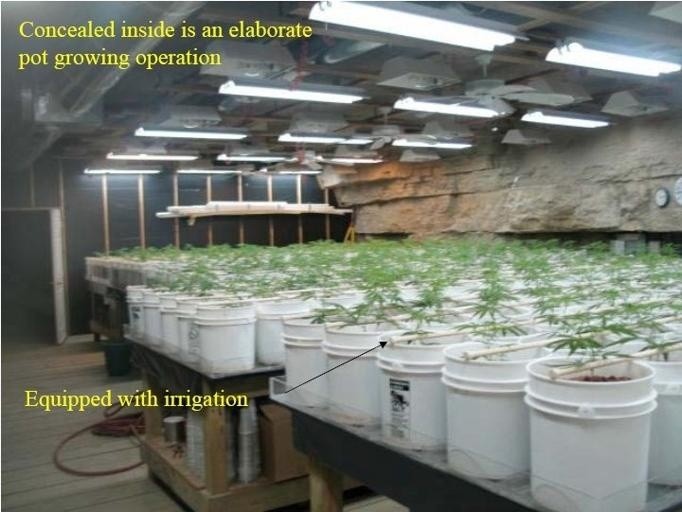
[630,341,682,485]
[195,302,255,373]
[523,355,658,511]
[325,322,406,423]
[374,329,474,452]
[159,294,179,346]
[254,301,319,366]
[81,231,681,512]
[440,340,553,481]
[125,284,164,336]
[176,297,198,352]
[279,316,346,409]
[142,291,160,343]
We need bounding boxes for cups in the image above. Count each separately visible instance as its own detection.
[163,415,186,442]
